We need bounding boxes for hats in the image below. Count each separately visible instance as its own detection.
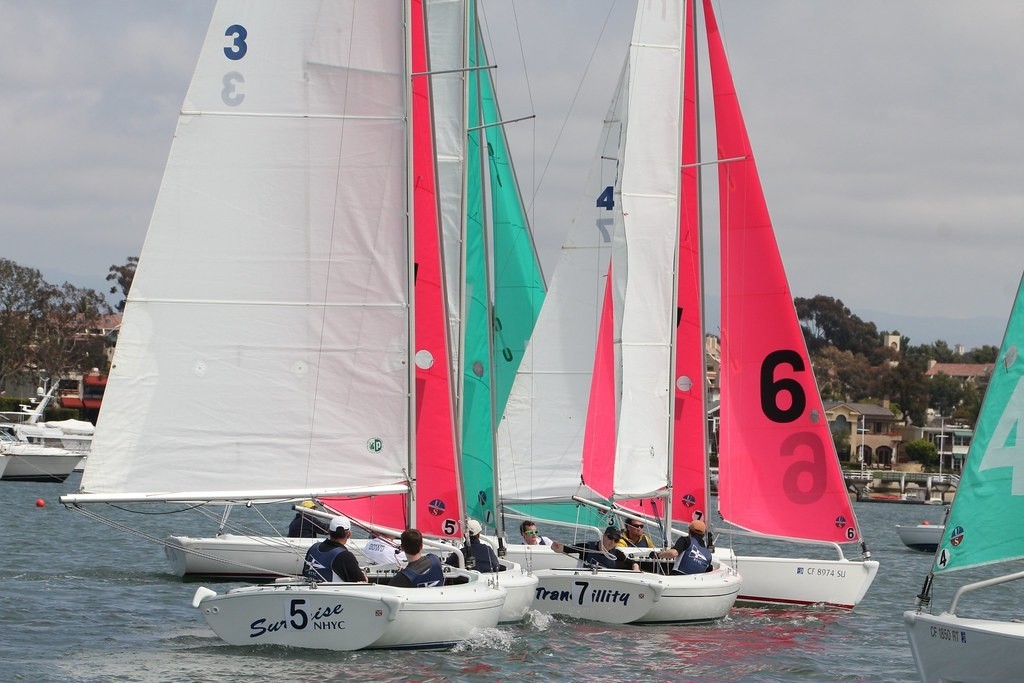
[606,525,621,538]
[330,516,351,531]
[464,520,483,537]
[688,520,705,537]
[300,500,318,512]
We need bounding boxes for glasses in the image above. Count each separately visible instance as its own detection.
[525,529,539,534]
[628,524,644,529]
[605,535,620,543]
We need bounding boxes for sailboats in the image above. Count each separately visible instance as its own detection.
[54,1,881,652]
[900,269,1024,683]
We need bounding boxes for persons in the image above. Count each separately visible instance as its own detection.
[551,526,641,573]
[649,520,714,576]
[520,520,554,545]
[444,520,499,573]
[388,529,445,588]
[288,500,332,538]
[363,533,401,565]
[613,514,657,548]
[302,515,368,586]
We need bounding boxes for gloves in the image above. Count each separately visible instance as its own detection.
[648,551,660,560]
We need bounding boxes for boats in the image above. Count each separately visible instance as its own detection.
[894,506,951,553]
[0,375,96,484]
